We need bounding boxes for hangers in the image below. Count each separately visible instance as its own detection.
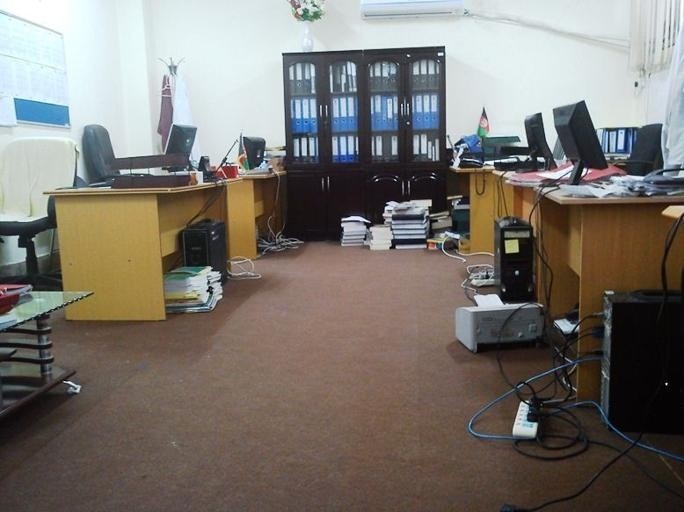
[150,73,185,98]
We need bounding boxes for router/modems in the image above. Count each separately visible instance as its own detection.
[553,319,579,335]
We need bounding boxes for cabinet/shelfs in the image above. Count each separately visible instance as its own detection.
[366,47,445,225]
[282,49,366,241]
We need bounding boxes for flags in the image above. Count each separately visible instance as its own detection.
[237,132,253,173]
[476,106,492,137]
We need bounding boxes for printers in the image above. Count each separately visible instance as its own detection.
[454,301,545,353]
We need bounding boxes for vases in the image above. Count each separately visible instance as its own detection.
[298,22,316,50]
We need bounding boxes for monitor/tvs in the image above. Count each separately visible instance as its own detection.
[553,100,608,185]
[163,123,198,171]
[243,136,266,170]
[523,112,558,172]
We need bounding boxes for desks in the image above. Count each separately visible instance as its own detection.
[1,291,93,418]
[494,176,684,406]
[450,158,500,255]
[43,178,242,321]
[214,170,285,259]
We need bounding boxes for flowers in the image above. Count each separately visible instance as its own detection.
[289,0,323,22]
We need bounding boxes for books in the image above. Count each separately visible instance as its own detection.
[163,261,224,315]
[336,200,467,249]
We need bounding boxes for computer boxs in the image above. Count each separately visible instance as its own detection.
[180,218,227,286]
[493,216,535,303]
[599,288,684,431]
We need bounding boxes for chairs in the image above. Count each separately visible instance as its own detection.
[622,124,664,174]
[83,124,125,181]
[1,138,77,287]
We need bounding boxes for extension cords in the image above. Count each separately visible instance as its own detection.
[512,400,543,439]
[469,278,495,288]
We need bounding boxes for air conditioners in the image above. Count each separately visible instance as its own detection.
[360,0,466,20]
